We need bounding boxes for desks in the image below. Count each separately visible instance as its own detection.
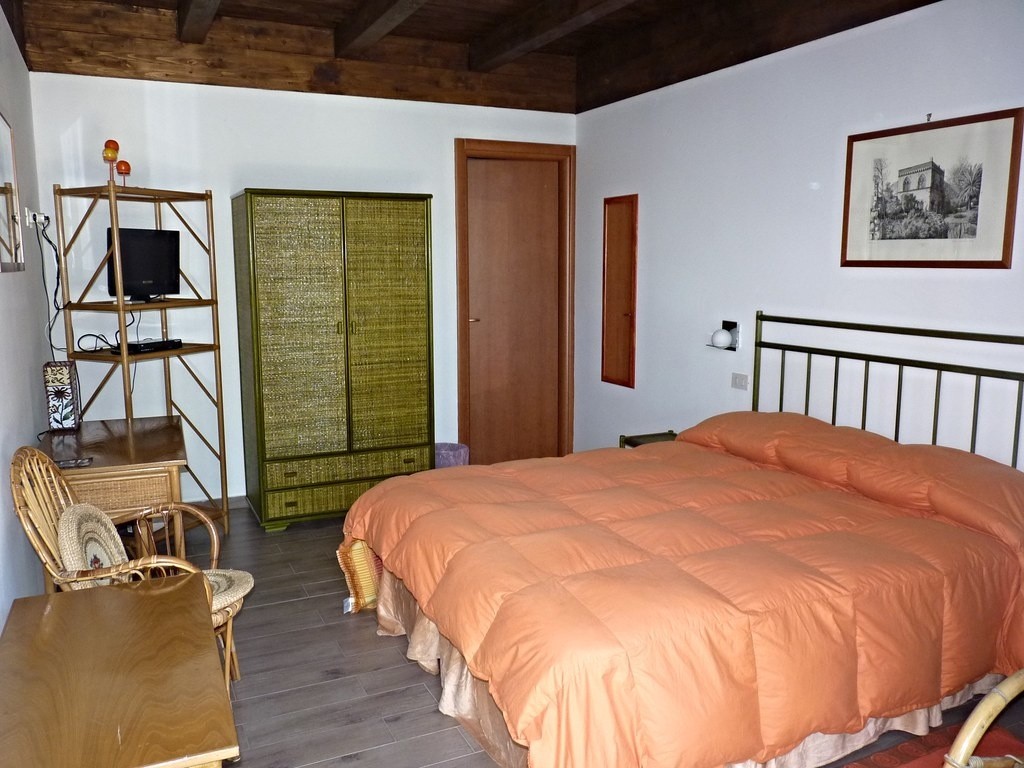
[0,572,244,768]
[30,415,192,570]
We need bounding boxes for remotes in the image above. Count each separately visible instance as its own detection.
[53,458,93,468]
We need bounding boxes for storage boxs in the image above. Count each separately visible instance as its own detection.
[43,358,80,431]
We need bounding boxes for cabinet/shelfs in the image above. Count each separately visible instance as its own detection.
[231,186,436,530]
[55,182,230,553]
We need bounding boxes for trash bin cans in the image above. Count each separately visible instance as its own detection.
[434,442,470,468]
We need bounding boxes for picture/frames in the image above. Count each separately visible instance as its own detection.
[841,105,1024,270]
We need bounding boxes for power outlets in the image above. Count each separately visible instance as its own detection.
[25,208,35,227]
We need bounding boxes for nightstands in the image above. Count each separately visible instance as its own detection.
[618,429,677,449]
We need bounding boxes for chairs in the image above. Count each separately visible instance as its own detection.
[940,668,1024,768]
[10,447,241,704]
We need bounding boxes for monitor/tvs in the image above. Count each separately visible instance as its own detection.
[107,227,180,304]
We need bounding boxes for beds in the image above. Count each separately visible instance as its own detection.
[336,313,1024,768]
